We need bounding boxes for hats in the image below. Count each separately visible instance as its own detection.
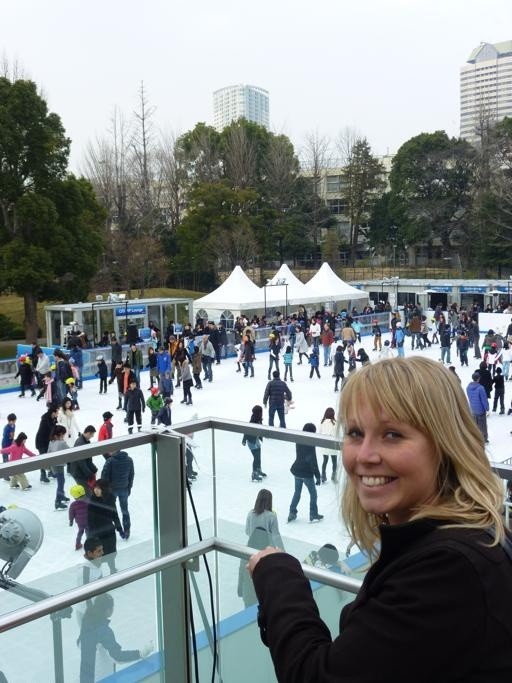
[336,346,344,351]
[488,330,495,334]
[253,406,261,414]
[103,412,113,421]
[495,367,501,373]
[168,335,174,340]
[472,372,479,379]
[158,346,164,351]
[25,357,30,364]
[235,344,241,349]
[313,348,318,355]
[207,321,214,324]
[357,349,363,355]
[269,334,274,338]
[437,302,442,306]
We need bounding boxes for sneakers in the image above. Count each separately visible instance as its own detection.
[41,478,49,482]
[188,480,192,484]
[192,472,197,475]
[62,496,70,501]
[310,515,322,520]
[15,484,19,487]
[55,500,67,508]
[189,476,196,479]
[252,473,263,481]
[26,485,32,488]
[288,515,297,521]
[258,471,266,477]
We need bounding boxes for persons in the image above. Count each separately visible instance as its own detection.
[338,298,389,351]
[391,302,431,358]
[475,361,492,417]
[237,490,286,608]
[491,367,505,415]
[431,301,484,367]
[482,329,498,368]
[493,333,506,358]
[344,344,356,372]
[146,317,231,489]
[317,407,342,484]
[466,372,490,446]
[356,348,369,367]
[281,394,295,417]
[230,306,338,381]
[484,298,512,314]
[263,370,292,428]
[506,318,512,364]
[448,366,461,385]
[287,422,324,523]
[247,354,512,683]
[301,544,339,581]
[334,346,346,391]
[494,341,512,383]
[242,405,267,483]
[485,342,503,379]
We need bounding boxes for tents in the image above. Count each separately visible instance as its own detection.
[192,262,370,329]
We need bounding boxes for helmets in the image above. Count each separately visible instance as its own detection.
[432,318,435,321]
[65,378,74,384]
[96,355,104,360]
[491,343,497,347]
[164,397,172,403]
[20,357,26,361]
[373,320,378,324]
[396,322,401,327]
[70,485,85,499]
[151,388,159,395]
[51,365,56,370]
